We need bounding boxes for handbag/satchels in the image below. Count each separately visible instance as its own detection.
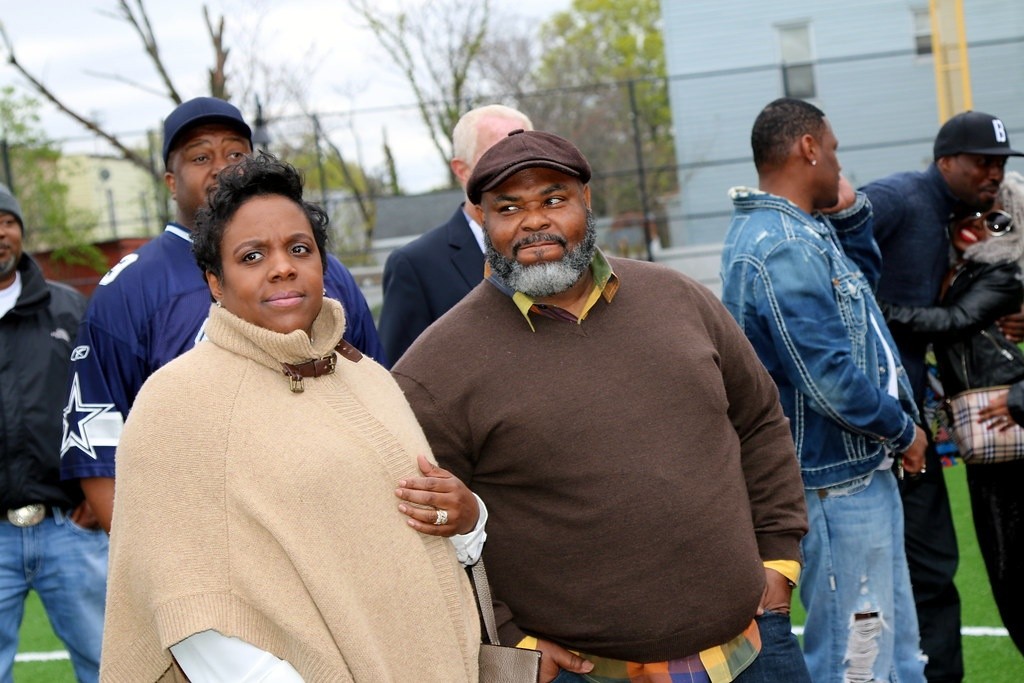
[946,383,1024,465]
[471,557,542,683]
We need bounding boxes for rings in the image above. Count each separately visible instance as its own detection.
[1001,414,1007,422]
[433,510,448,525]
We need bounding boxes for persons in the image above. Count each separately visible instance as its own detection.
[0,182,109,683]
[878,171,1024,659]
[376,104,535,368]
[854,109,1024,683]
[58,96,386,539]
[98,147,487,683]
[389,129,813,683]
[977,381,1024,432]
[716,98,930,683]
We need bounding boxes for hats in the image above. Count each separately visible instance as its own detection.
[162,97,254,166]
[933,110,1024,161]
[466,128,592,204]
[0,183,24,238]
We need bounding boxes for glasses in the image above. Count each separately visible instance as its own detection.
[962,205,1015,236]
